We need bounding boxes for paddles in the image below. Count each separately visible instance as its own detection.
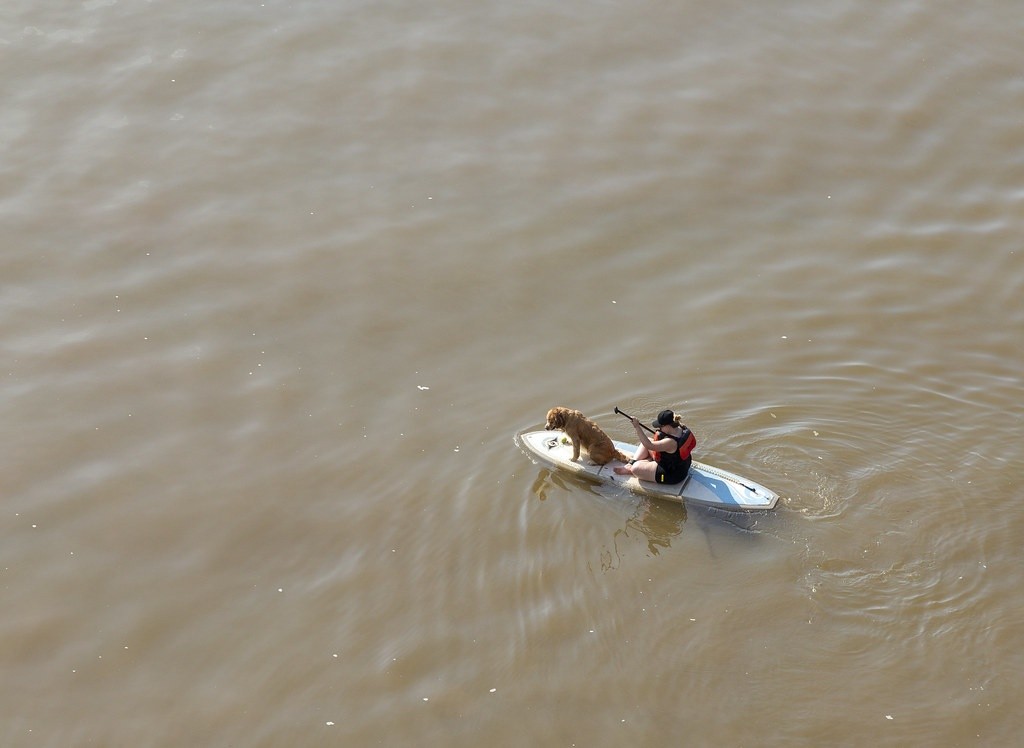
[613,405,655,434]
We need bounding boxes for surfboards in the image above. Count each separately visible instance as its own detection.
[519,430,780,511]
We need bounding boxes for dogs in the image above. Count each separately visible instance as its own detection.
[544,407,632,466]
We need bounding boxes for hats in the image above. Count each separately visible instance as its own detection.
[652,410,673,428]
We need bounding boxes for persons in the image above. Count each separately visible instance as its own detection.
[613,409,696,485]
